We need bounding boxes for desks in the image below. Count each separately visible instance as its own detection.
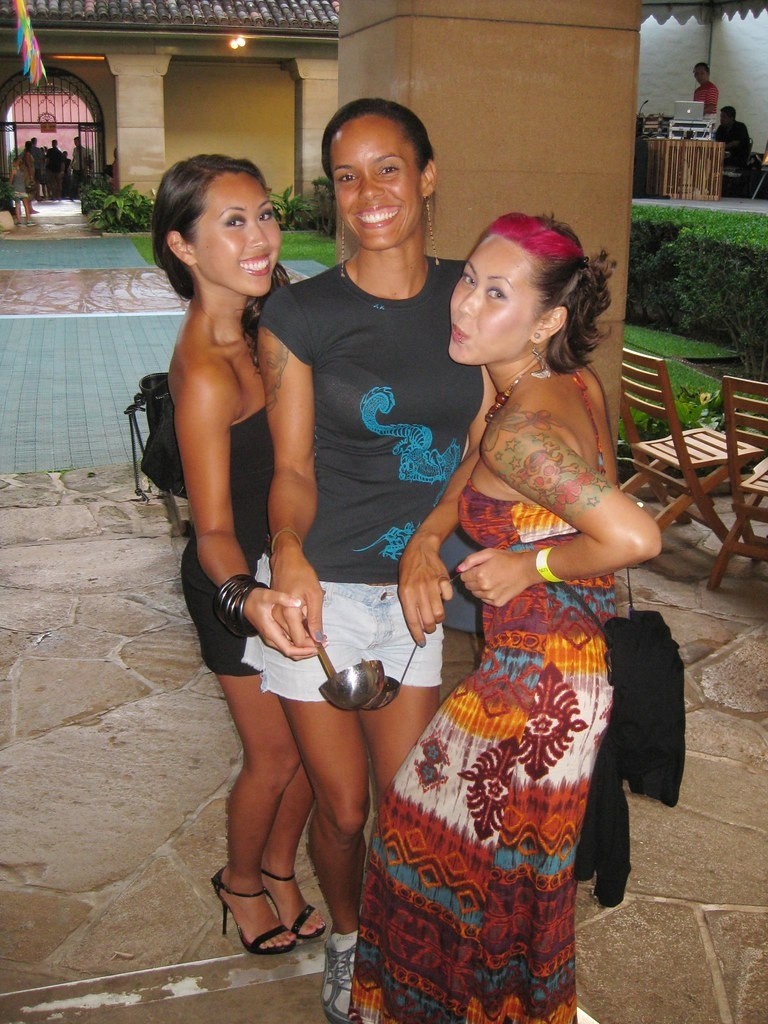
[635,137,725,202]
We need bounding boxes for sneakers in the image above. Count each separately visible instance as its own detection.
[321,924,359,1024]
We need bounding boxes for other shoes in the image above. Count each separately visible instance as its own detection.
[26,221,37,226]
[17,222,22,227]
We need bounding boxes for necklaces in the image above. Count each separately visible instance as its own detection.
[483,372,524,422]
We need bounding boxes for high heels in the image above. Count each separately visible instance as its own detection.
[260,869,326,939]
[211,865,296,954]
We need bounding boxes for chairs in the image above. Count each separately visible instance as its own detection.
[617,347,763,542]
[722,137,768,198]
[706,376,768,591]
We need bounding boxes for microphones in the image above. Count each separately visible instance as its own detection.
[639,100,649,116]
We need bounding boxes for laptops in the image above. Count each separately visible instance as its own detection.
[674,101,704,121]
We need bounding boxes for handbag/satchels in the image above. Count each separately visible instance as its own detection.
[140,372,188,497]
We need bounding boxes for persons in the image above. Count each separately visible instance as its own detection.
[257,97,497,1022]
[9,137,87,227]
[714,106,750,167]
[153,156,329,955]
[349,206,661,1024]
[692,63,718,130]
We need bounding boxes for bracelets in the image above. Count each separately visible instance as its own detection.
[271,527,302,552]
[212,574,266,637]
[536,545,565,582]
